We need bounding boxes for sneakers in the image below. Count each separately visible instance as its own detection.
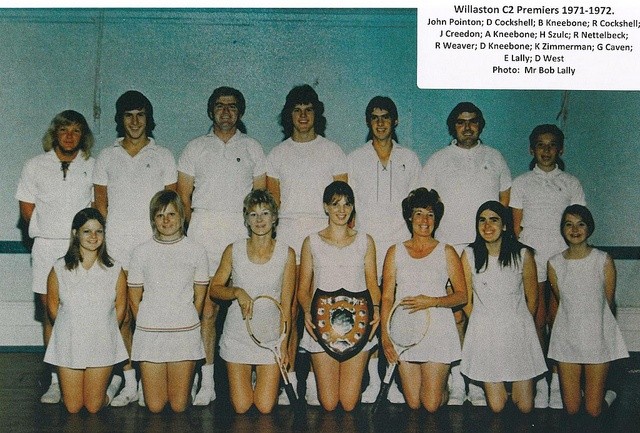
[193,364,217,405]
[40,372,64,404]
[138,377,146,407]
[361,357,382,403]
[106,374,122,407]
[534,374,550,408]
[447,361,467,405]
[549,372,564,409]
[278,371,299,405]
[605,390,617,408]
[304,371,321,406]
[467,379,487,406]
[111,368,139,407]
[191,372,199,404]
[385,366,406,404]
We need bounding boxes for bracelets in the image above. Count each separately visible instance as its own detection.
[372,304,380,307]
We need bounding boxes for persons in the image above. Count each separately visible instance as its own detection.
[346,94,421,403]
[459,199,540,415]
[540,203,632,419]
[508,124,587,410]
[420,98,513,407]
[127,189,211,413]
[46,207,127,415]
[264,82,347,407]
[293,179,381,413]
[209,188,297,416]
[176,84,267,407]
[16,109,97,405]
[92,90,180,410]
[380,185,469,414]
